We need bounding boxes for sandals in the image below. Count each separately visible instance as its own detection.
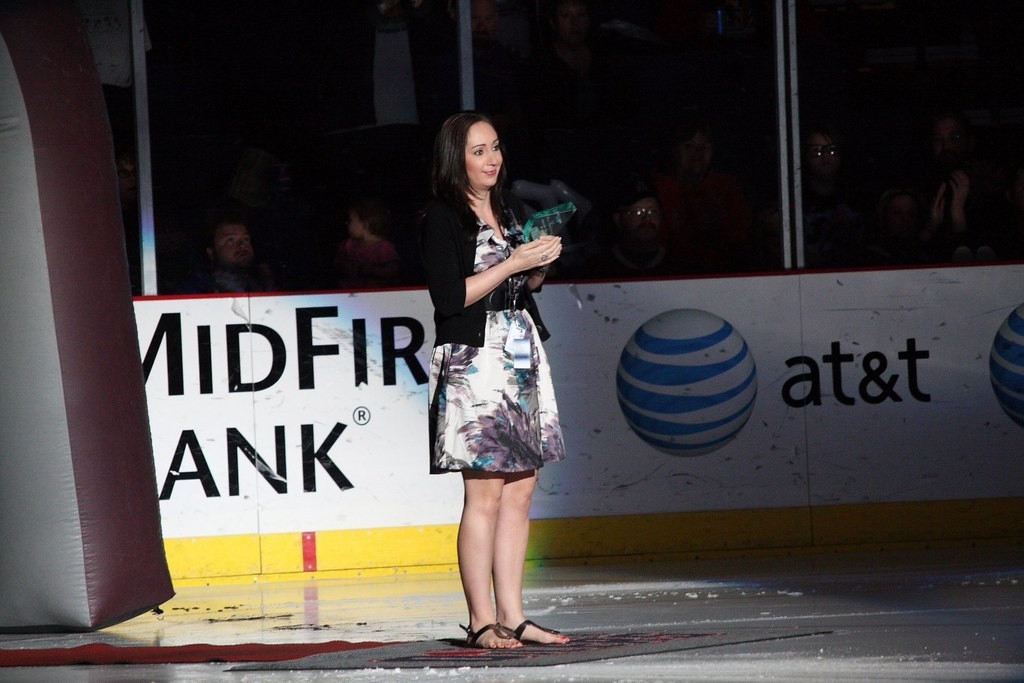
[497,620,566,646]
[463,621,520,648]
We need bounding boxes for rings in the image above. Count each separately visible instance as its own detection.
[541,252,546,262]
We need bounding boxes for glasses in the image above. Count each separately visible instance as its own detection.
[804,143,844,157]
[618,207,660,217]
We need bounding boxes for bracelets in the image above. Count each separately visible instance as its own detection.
[536,265,550,272]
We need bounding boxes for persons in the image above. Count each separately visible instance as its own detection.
[413,112,574,648]
[150,0,1024,291]
